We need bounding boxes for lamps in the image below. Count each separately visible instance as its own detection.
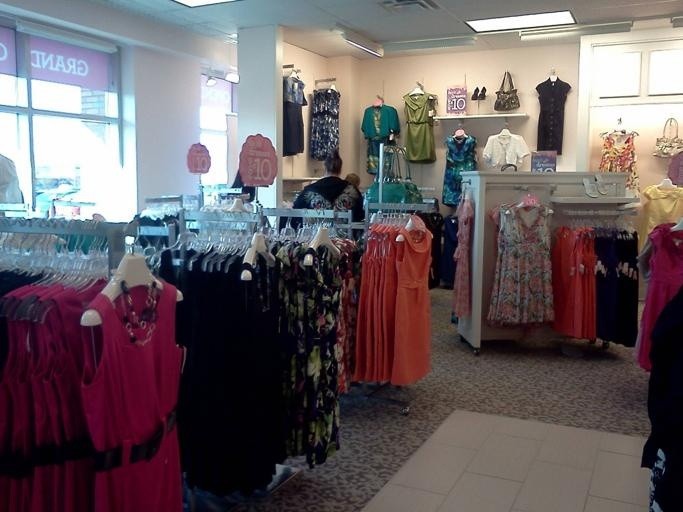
[464,10,577,34]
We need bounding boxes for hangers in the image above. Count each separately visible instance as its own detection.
[391,204,409,241]
[287,63,302,82]
[0,216,109,323]
[88,216,152,266]
[396,204,434,242]
[188,219,206,272]
[667,213,683,235]
[226,217,255,274]
[138,194,180,220]
[655,177,679,191]
[189,194,255,217]
[241,214,276,281]
[217,226,239,273]
[378,204,401,242]
[401,82,435,100]
[372,96,384,110]
[497,121,513,143]
[209,221,228,275]
[150,226,167,266]
[384,204,399,242]
[505,185,554,216]
[602,118,639,137]
[546,65,559,84]
[326,76,338,94]
[556,209,641,241]
[265,208,296,245]
[599,117,622,135]
[296,209,312,245]
[80,221,183,329]
[304,209,340,267]
[306,209,320,246]
[156,224,181,269]
[368,204,383,242]
[372,201,386,243]
[201,218,228,274]
[443,123,478,143]
[495,184,524,207]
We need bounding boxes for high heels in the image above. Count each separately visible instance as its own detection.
[581,178,598,198]
[595,171,610,196]
[471,87,479,101]
[478,86,486,100]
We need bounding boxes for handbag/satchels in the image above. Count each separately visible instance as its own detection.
[653,117,683,159]
[362,147,409,215]
[390,148,425,205]
[493,69,522,111]
